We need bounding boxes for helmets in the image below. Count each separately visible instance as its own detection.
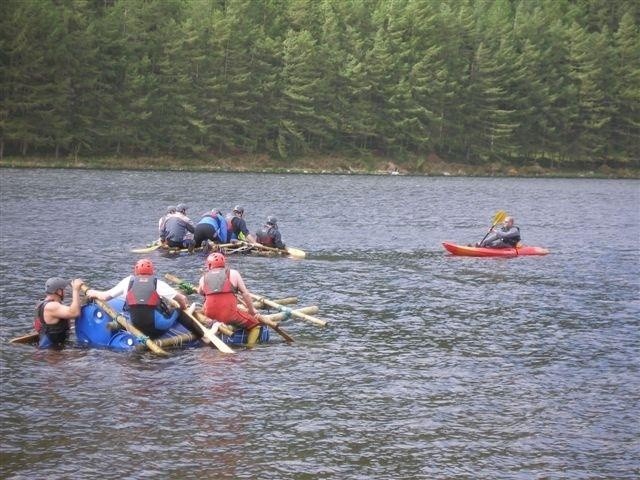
[45,277,68,294]
[166,204,277,227]
[134,259,154,275]
[207,253,225,269]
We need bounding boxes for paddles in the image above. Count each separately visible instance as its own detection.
[235,294,296,342]
[130,243,160,253]
[241,240,306,259]
[479,210,508,247]
[9,326,76,345]
[169,298,236,353]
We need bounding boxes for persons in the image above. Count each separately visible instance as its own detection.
[225,204,263,243]
[160,203,195,248]
[198,253,262,349]
[256,215,288,252]
[187,207,228,255]
[33,275,84,352]
[158,205,177,245]
[84,258,187,336]
[476,216,521,249]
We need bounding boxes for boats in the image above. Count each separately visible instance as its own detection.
[441,239,550,259]
[157,232,307,260]
[73,279,330,354]
